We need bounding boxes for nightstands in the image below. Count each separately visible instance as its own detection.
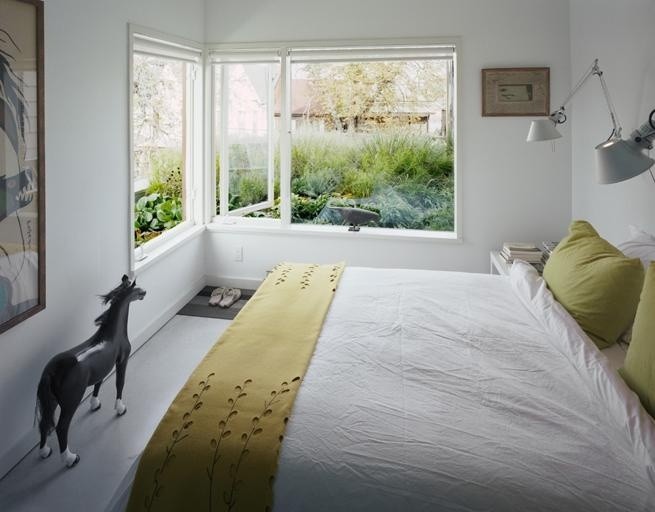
[488,251,513,275]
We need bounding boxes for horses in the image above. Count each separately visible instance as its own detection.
[33,274,147,468]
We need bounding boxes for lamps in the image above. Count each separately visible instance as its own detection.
[527,59,655,184]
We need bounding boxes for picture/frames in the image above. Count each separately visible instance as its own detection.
[0,0,47,335]
[481,68,550,117]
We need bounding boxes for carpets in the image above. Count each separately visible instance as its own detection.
[177,285,258,320]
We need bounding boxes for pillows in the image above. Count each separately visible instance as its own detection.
[541,222,647,347]
[600,224,655,347]
[621,262,655,415]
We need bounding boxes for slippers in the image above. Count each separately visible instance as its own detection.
[208,286,228,307]
[219,287,241,308]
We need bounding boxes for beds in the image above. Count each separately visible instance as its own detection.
[126,229,655,512]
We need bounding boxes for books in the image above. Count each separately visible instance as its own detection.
[498,241,562,273]
